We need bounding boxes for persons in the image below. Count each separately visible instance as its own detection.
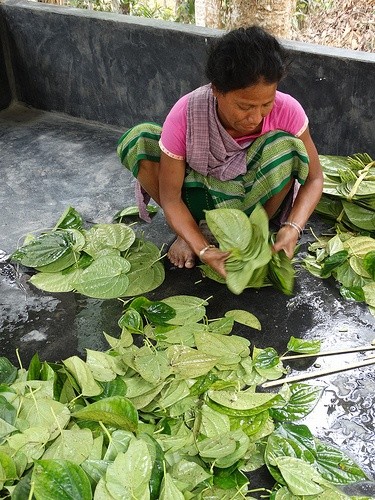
[115,25,326,270]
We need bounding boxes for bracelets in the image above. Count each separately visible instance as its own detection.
[199,243,216,266]
[279,218,303,241]
